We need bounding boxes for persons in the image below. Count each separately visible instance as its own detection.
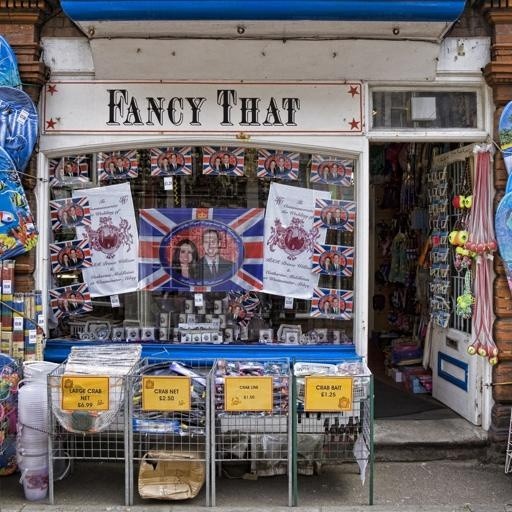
[56,147,349,321]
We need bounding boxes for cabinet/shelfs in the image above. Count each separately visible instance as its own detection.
[375,209,396,333]
[136,173,355,345]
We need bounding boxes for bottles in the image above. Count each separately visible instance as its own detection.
[278,305,288,325]
[320,416,362,464]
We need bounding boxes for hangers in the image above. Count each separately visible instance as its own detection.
[391,227,406,248]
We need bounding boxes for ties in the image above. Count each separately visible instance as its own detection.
[120,163,284,174]
[71,173,77,309]
[335,173,340,313]
[211,260,217,282]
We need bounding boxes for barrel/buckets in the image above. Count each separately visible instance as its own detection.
[14,357,61,502]
